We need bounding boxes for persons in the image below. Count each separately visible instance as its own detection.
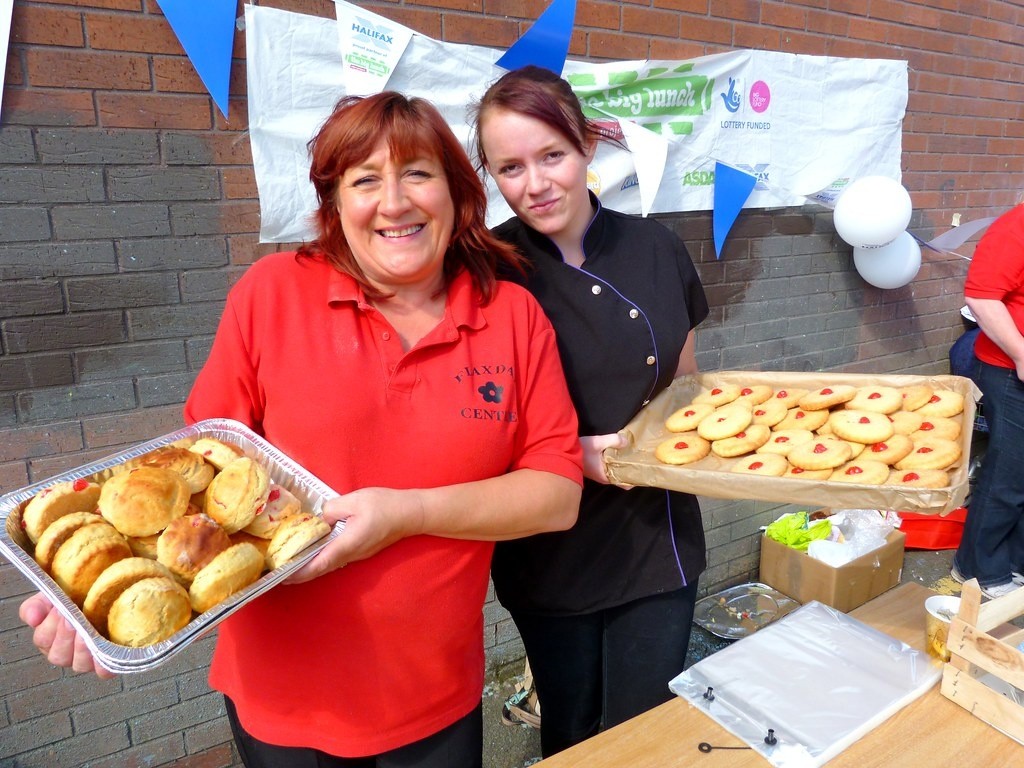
[465,62,711,759]
[952,199,1024,598]
[18,87,583,768]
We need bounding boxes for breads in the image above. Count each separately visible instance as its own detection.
[24,438,331,647]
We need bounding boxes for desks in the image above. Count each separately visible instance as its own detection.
[525,581,1024,768]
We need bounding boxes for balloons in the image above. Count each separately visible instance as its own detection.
[834,174,912,249]
[854,230,922,289]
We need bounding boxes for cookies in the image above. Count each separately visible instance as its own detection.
[656,385,964,489]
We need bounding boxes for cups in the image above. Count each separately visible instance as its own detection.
[922,595,962,662]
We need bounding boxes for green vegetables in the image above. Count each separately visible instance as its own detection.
[766,513,830,551]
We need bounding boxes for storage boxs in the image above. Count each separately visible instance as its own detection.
[899,507,967,550]
[758,508,906,614]
